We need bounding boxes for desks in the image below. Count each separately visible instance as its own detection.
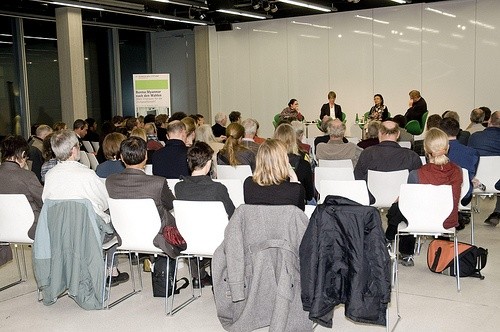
[353,123,382,140]
[302,121,320,138]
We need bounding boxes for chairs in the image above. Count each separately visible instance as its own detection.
[0,111,500,332]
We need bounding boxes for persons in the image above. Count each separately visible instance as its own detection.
[368,94,390,120]
[280,99,304,123]
[320,91,343,123]
[403,90,429,129]
[0,107,500,299]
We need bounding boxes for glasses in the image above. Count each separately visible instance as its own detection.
[71,143,82,150]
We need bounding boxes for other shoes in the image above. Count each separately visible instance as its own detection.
[459,211,470,224]
[484,211,500,226]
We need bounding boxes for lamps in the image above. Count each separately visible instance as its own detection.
[251,0,278,13]
[190,5,206,20]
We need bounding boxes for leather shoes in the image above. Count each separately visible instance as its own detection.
[106,269,128,287]
[193,274,213,289]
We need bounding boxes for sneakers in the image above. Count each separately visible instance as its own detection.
[384,238,392,253]
[396,253,414,266]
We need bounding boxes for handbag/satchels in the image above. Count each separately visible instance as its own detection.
[152,256,177,298]
[427,240,488,280]
